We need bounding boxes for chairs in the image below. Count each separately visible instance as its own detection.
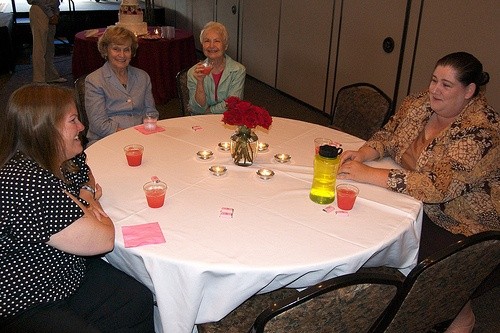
[74,61,499,333]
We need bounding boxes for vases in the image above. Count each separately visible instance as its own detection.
[230,125,259,165]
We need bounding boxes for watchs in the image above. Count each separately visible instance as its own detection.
[82,185,94,193]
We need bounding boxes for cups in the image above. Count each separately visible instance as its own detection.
[142,180,167,208]
[336,183,359,211]
[124,143,144,167]
[314,137,333,154]
[161,26,175,40]
[143,111,159,131]
[200,60,215,75]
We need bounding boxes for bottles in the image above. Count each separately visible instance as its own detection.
[309,145,343,205]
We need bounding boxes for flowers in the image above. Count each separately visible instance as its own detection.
[223,97,273,131]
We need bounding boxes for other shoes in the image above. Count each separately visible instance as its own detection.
[444,311,475,333]
[46,77,68,83]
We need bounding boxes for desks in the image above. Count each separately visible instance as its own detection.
[0,13,14,49]
[86,114,425,333]
[72,24,195,106]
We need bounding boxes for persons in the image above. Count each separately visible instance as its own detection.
[0,83,156,333]
[27,0,67,84]
[337,52,500,333]
[187,22,246,116]
[85,26,159,149]
